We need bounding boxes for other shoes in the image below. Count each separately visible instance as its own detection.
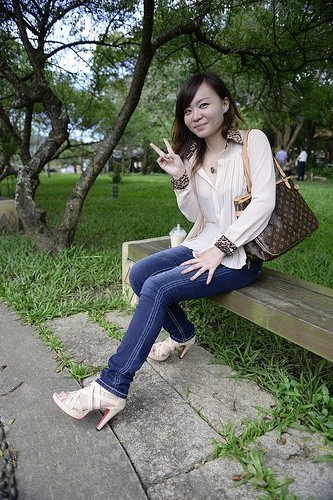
[296,178,299,181]
[301,179,304,181]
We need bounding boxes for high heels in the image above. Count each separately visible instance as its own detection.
[148,336,195,361]
[52,381,126,431]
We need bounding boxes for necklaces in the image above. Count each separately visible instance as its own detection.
[211,167,215,174]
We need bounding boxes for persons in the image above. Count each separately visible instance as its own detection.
[53,71,319,430]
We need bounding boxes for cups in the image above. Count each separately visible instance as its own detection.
[169,227,187,248]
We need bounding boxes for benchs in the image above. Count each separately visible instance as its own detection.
[121,235,333,363]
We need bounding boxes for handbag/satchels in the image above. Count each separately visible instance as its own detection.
[234,128,320,262]
